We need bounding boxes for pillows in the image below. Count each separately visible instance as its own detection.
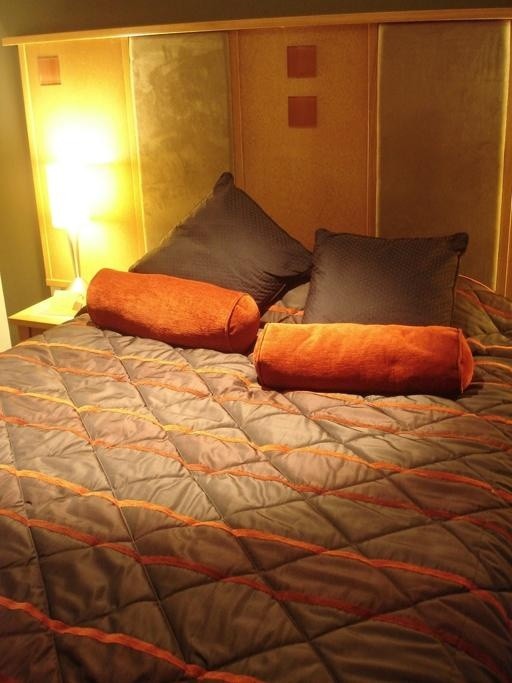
[129,170,315,311]
[303,228,470,326]
[254,321,475,394]
[87,269,261,354]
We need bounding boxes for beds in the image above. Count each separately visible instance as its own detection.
[0,278,511,683]
